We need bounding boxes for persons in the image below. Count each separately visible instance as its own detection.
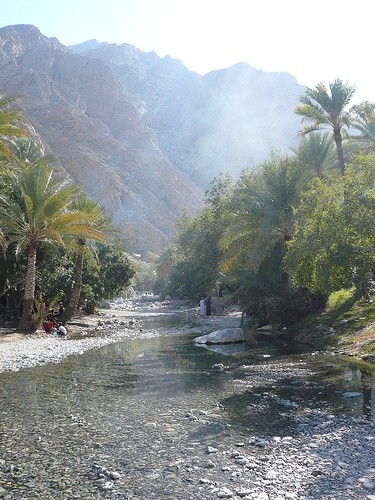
[206,297,211,315]
[200,297,205,316]
[33,299,68,335]
